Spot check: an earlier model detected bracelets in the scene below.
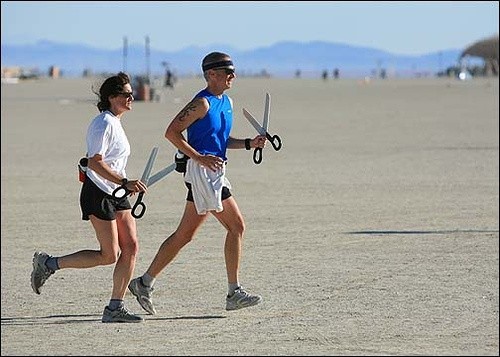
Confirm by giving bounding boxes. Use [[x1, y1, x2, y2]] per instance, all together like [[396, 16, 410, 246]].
[[244, 138, 250, 150]]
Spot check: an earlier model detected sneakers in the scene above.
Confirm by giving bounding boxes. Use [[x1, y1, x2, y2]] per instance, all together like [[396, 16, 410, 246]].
[[226, 285, 262, 311], [102, 301, 144, 322], [31, 250, 55, 295], [128, 277, 156, 315]]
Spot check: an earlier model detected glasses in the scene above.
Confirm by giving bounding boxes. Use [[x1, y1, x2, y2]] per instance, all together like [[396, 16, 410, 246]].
[[213, 68, 235, 75], [116, 92, 133, 98]]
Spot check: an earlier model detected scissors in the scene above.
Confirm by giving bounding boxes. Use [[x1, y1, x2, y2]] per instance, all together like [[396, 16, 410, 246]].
[[111, 147, 177, 219], [243, 93, 282, 164]]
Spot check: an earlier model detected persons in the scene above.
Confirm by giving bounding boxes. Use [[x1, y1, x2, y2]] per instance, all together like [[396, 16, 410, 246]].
[[30, 73, 147, 324], [127, 53, 266, 315]]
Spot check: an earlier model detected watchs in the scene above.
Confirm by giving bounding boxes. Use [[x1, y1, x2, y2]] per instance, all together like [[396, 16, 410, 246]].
[[122, 178, 127, 188]]
